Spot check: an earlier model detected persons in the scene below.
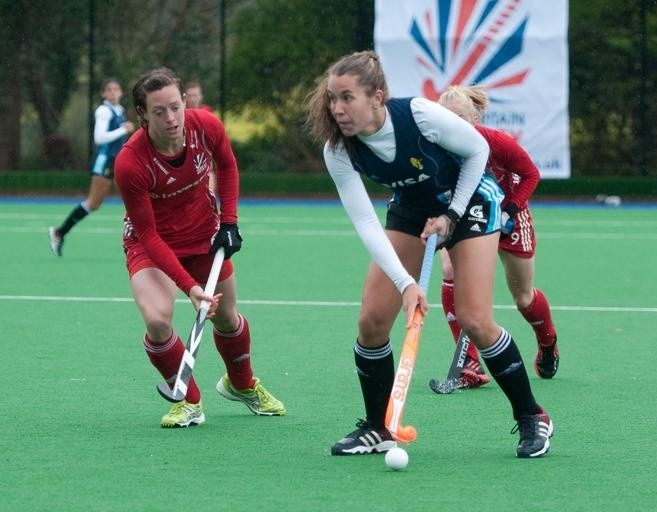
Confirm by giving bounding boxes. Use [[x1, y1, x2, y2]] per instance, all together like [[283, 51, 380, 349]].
[[304, 50, 554, 458], [184, 81, 217, 197], [48, 78, 135, 257], [114, 67, 286, 428], [435, 86, 560, 389]]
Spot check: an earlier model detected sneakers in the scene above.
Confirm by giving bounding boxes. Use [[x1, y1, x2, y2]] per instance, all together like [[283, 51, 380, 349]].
[[461, 356, 490, 388], [159, 397, 205, 428], [511, 407, 554, 454], [48, 226, 63, 257], [534, 333, 559, 379], [331, 419, 398, 456], [216, 372, 286, 415]]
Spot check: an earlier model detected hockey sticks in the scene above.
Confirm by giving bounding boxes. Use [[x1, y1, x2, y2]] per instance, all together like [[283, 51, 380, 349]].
[[384, 234, 437, 442], [154, 248, 226, 402], [429, 329, 470, 395]]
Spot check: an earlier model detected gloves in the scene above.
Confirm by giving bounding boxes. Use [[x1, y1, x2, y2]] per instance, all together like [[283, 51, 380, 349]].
[[501, 202, 519, 241], [209, 222, 243, 260]]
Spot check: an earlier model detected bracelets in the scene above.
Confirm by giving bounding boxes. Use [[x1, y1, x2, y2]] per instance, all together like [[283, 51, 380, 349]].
[[501, 201, 519, 219]]
